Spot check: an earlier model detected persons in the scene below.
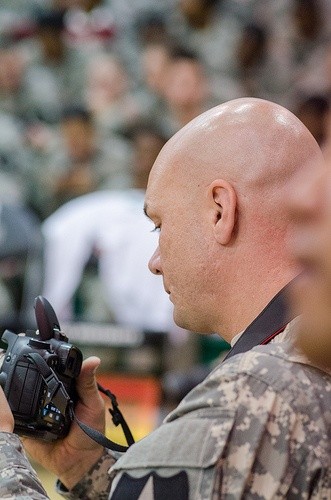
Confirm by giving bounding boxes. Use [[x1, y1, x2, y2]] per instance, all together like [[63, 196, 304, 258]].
[[0, 0, 331, 393], [0, 94, 331, 500]]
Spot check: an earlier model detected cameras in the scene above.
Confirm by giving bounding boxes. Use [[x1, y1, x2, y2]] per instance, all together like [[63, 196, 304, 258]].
[[0, 295, 83, 442]]
